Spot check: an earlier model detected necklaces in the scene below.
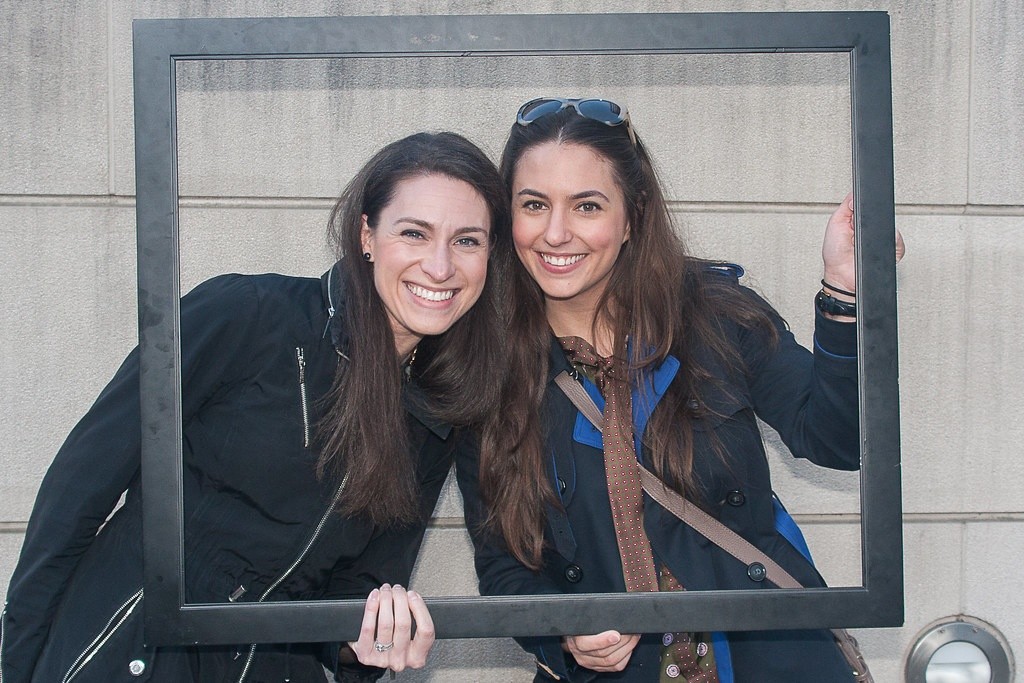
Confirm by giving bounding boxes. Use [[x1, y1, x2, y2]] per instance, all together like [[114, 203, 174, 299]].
[[408, 346, 418, 365]]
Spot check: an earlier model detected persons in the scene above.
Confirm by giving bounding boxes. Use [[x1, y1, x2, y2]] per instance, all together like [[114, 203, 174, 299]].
[[454, 98, 907, 683], [1, 129, 522, 683]]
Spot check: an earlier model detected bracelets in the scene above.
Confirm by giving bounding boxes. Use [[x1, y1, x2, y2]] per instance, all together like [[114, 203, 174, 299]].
[[816, 280, 859, 318]]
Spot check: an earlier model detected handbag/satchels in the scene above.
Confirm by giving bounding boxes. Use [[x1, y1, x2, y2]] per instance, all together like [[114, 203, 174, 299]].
[[830, 629, 875, 683]]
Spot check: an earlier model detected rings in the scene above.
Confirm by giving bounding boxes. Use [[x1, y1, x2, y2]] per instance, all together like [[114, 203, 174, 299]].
[[375, 638, 393, 652]]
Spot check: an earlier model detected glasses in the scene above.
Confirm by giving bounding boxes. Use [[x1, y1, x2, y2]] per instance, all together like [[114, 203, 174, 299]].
[[516, 97, 637, 152]]
